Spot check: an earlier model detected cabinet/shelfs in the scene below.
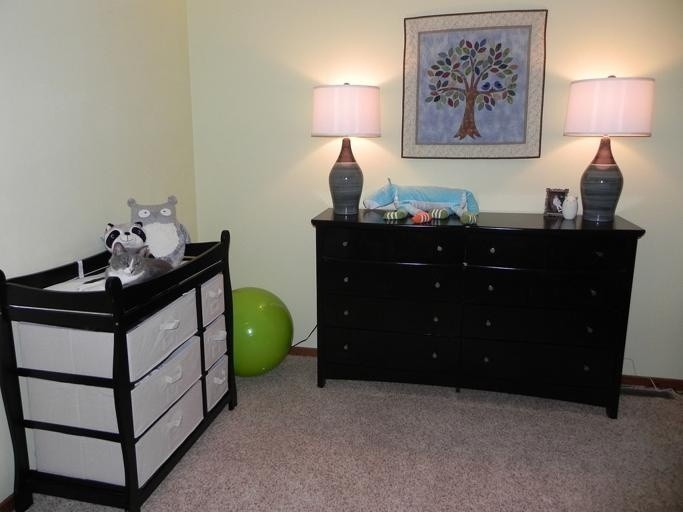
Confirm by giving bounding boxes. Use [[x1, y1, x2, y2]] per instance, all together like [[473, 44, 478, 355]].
[[313, 208, 464, 399], [0, 230, 238, 512], [465, 207, 647, 420]]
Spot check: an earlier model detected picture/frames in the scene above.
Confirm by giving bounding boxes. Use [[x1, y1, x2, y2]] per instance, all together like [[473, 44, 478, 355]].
[[401, 9, 548, 158], [544, 187, 568, 216]]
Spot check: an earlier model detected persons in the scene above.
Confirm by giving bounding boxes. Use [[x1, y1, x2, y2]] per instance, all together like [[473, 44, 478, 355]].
[[552, 194, 562, 212]]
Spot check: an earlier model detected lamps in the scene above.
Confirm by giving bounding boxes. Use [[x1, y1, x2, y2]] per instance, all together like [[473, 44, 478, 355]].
[[310, 83, 382, 215], [563, 74, 656, 222]]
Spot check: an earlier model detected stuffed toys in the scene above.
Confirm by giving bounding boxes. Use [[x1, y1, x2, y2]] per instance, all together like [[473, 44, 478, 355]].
[[363, 176, 479, 225], [128, 195, 190, 266], [103, 221, 155, 259]]
